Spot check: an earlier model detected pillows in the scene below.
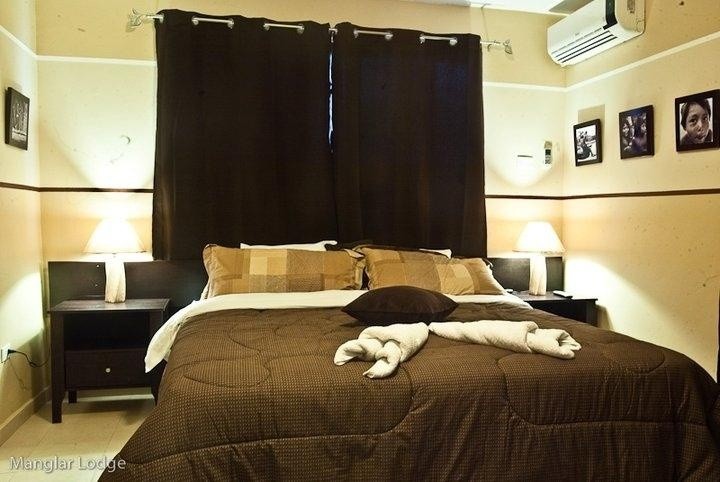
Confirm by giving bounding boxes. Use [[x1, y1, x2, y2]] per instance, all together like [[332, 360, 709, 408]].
[[323, 238, 454, 261], [235, 238, 342, 253], [339, 284, 461, 327], [354, 242, 505, 299], [200, 240, 365, 297]]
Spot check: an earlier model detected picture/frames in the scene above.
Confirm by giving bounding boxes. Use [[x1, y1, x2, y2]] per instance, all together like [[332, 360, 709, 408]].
[[571, 116, 603, 166], [619, 104, 654, 160], [5, 86, 30, 153], [674, 88, 719, 154]]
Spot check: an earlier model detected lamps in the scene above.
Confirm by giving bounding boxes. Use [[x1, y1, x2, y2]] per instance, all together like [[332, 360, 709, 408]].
[[83, 219, 149, 303], [511, 219, 568, 294]]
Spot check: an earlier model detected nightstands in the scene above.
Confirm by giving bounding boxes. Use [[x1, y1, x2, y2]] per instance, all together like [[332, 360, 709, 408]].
[[501, 283, 600, 327], [43, 295, 171, 427]]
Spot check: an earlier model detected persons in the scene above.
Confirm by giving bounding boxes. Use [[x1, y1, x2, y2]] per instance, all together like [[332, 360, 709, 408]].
[[621, 113, 647, 154], [679, 99, 713, 144], [574, 128, 596, 161]]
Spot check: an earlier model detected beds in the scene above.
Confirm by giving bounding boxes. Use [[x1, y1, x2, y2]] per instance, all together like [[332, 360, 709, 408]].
[[45, 238, 720, 482]]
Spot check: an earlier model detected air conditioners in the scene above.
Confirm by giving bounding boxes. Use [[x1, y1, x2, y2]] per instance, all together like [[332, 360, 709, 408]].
[[544, 0, 646, 70]]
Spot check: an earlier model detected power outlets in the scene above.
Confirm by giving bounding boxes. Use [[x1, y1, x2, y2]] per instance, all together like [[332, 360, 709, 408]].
[[0, 344, 10, 363]]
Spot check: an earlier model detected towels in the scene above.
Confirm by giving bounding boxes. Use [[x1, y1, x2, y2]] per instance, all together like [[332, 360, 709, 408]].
[[332, 320, 432, 384], [429, 318, 585, 362]]
